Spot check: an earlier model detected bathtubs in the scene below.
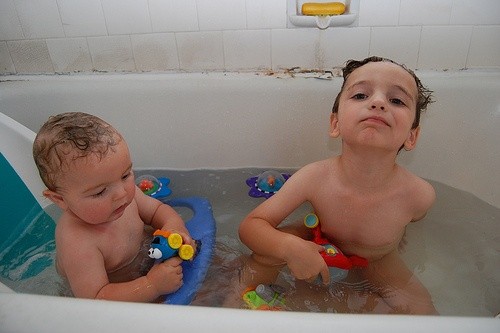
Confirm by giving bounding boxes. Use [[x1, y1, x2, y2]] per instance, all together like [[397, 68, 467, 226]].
[[0, 68, 499, 333]]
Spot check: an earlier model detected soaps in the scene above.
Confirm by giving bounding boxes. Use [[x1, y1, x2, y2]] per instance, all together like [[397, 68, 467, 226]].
[[302, 3, 346, 15]]
